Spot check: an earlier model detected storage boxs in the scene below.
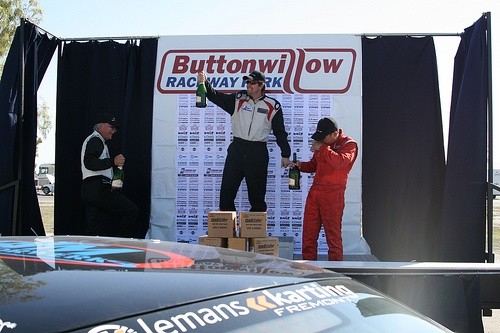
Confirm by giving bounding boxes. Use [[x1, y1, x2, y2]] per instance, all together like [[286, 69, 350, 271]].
[[199, 210, 278, 258]]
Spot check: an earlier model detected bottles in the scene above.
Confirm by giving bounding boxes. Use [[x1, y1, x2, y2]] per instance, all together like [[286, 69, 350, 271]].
[[288, 152, 300, 189], [196, 71, 207, 108], [111, 165, 125, 192]]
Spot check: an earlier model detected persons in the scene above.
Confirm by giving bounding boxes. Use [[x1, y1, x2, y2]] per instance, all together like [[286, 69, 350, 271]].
[[289, 117, 358, 261], [80, 112, 139, 234], [197, 71, 291, 252]]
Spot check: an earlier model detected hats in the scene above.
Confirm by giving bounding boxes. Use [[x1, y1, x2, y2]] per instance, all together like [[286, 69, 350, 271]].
[[91, 111, 121, 127], [311, 116, 338, 141], [243, 70, 265, 84]]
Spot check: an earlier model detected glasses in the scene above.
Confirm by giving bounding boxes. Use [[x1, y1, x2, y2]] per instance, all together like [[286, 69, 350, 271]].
[[246, 80, 259, 85]]
